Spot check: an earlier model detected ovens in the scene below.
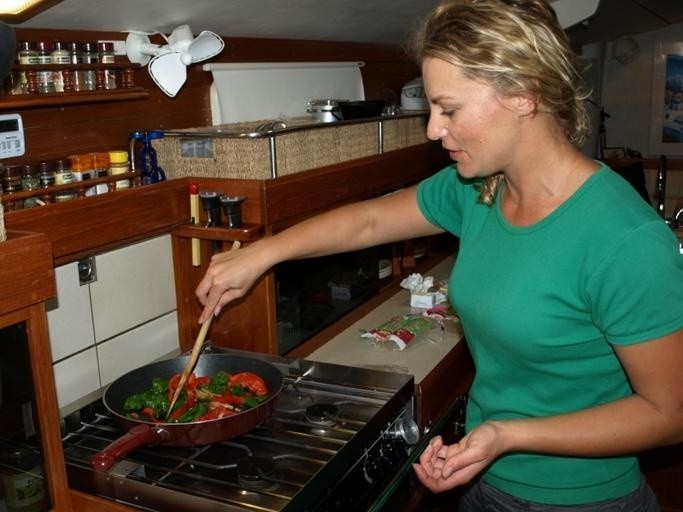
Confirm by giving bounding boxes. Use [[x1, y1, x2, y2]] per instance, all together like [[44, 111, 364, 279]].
[[306, 393, 466, 512]]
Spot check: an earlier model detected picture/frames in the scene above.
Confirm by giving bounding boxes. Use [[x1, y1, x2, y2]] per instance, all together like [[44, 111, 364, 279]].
[[601, 145, 626, 161], [645, 40, 683, 156]]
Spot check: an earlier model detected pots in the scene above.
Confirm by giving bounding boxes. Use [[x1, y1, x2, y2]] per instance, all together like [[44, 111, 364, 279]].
[[88, 353, 284, 472], [330, 100, 386, 121], [306, 99, 349, 123]]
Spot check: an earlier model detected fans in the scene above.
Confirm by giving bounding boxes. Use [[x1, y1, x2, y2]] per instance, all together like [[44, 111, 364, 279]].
[[118, 23, 224, 99]]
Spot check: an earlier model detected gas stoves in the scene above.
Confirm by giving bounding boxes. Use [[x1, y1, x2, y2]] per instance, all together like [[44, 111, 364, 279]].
[[24, 340, 413, 512]]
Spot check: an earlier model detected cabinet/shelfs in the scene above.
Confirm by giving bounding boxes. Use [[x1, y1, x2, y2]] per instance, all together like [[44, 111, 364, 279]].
[[0, 64, 190, 267], [1, 232, 71, 510], [171, 139, 458, 359], [417, 337, 477, 511]]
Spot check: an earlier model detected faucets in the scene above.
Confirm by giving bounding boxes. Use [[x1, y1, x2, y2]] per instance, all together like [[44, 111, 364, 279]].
[[655, 153, 675, 229]]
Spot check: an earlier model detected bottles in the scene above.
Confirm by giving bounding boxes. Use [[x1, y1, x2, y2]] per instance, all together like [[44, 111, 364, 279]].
[[3, 40, 134, 96], [0, 148, 131, 212], [0, 445, 49, 512]]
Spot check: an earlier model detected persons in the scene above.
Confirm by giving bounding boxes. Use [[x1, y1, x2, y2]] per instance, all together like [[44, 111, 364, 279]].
[[192, 0, 683, 511]]
